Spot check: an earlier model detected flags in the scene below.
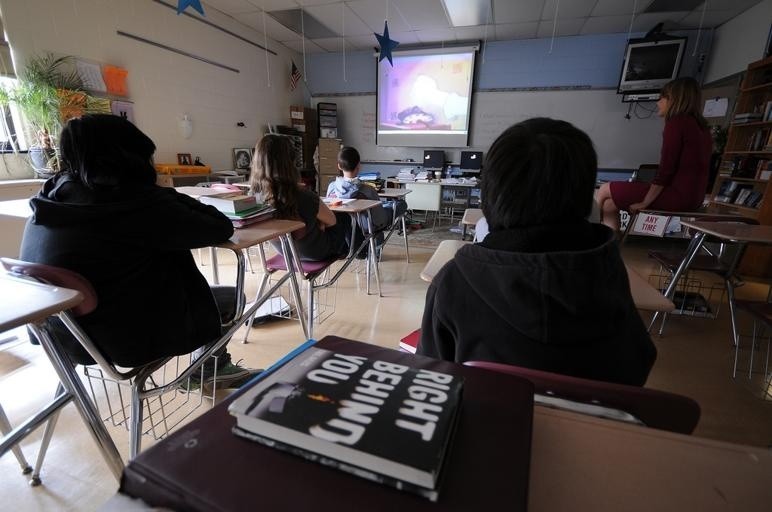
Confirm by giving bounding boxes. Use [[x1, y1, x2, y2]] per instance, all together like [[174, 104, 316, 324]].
[[290, 61, 301, 92]]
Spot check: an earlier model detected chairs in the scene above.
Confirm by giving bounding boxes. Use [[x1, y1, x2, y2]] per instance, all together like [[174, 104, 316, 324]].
[[734, 287, 769, 382], [0, 175, 772, 300], [462, 361, 700, 431], [240, 232, 349, 341], [0, 258, 218, 486]]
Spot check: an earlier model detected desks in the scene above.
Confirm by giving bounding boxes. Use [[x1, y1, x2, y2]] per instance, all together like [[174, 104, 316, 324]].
[[153, 163, 211, 188], [0, 273, 123, 485], [420, 238, 675, 334], [228, 219, 307, 339], [100, 405, 772, 510]]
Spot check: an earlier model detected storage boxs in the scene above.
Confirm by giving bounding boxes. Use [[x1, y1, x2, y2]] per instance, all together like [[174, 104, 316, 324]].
[[290, 104, 316, 120], [291, 120, 317, 134], [319, 138, 343, 175], [300, 134, 315, 143]]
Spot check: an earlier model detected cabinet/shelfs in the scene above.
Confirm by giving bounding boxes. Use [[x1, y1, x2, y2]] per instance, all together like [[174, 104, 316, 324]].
[[710, 54, 772, 281]]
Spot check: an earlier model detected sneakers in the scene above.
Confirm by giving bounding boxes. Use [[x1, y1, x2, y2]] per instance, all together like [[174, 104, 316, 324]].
[[177, 359, 264, 394]]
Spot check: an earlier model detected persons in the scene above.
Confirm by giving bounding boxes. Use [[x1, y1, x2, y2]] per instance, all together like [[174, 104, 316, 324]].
[[247, 133, 369, 263], [326, 146, 393, 262], [416, 117, 658, 387], [596, 77, 713, 240], [20, 113, 265, 393], [237, 151, 250, 171]]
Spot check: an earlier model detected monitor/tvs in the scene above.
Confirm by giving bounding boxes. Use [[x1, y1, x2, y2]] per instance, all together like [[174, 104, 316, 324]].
[[460, 150, 484, 174], [616, 36, 689, 93], [424, 149, 445, 170]]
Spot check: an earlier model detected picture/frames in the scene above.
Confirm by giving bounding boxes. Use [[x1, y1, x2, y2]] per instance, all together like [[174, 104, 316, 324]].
[[232, 147, 253, 171], [177, 153, 193, 165]]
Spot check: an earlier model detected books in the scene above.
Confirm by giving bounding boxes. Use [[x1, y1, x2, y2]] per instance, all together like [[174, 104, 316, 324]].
[[400, 328, 421, 354], [396, 168, 432, 184], [227, 347, 466, 504], [198, 193, 277, 229], [713, 100, 772, 208]]
